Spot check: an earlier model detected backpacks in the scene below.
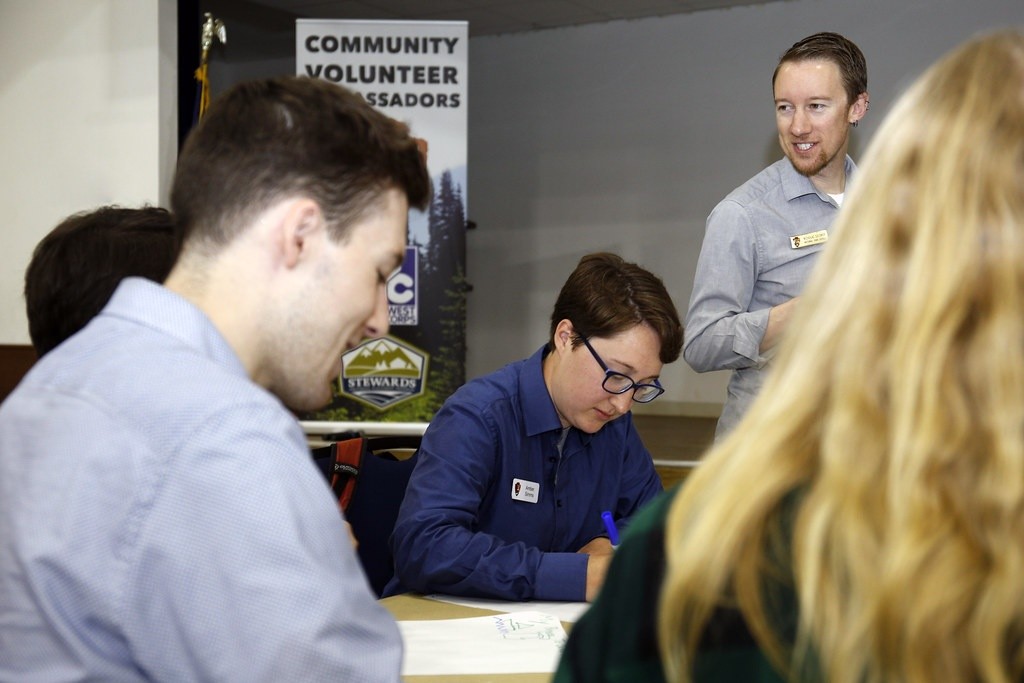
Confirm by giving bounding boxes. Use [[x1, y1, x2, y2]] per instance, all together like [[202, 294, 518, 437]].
[[310, 439, 419, 600]]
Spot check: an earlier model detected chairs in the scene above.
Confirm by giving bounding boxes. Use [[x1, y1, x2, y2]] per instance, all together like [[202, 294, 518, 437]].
[[313, 436, 423, 598]]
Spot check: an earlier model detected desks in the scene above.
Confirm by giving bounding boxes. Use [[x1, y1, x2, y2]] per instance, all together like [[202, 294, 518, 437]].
[[376, 593, 591, 683]]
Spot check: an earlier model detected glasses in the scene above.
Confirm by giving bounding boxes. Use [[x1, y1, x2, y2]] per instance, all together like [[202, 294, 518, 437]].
[[584, 339, 665, 403]]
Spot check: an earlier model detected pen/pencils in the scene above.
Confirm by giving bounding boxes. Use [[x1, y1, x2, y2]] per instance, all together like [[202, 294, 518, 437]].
[[601, 511, 621, 552]]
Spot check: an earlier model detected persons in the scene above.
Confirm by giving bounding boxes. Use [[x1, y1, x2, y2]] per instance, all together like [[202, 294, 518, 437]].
[[23, 207, 181, 356], [0, 77, 429, 683], [382, 253, 685, 602], [681, 32, 869, 445], [552, 20, 1024, 683]]
